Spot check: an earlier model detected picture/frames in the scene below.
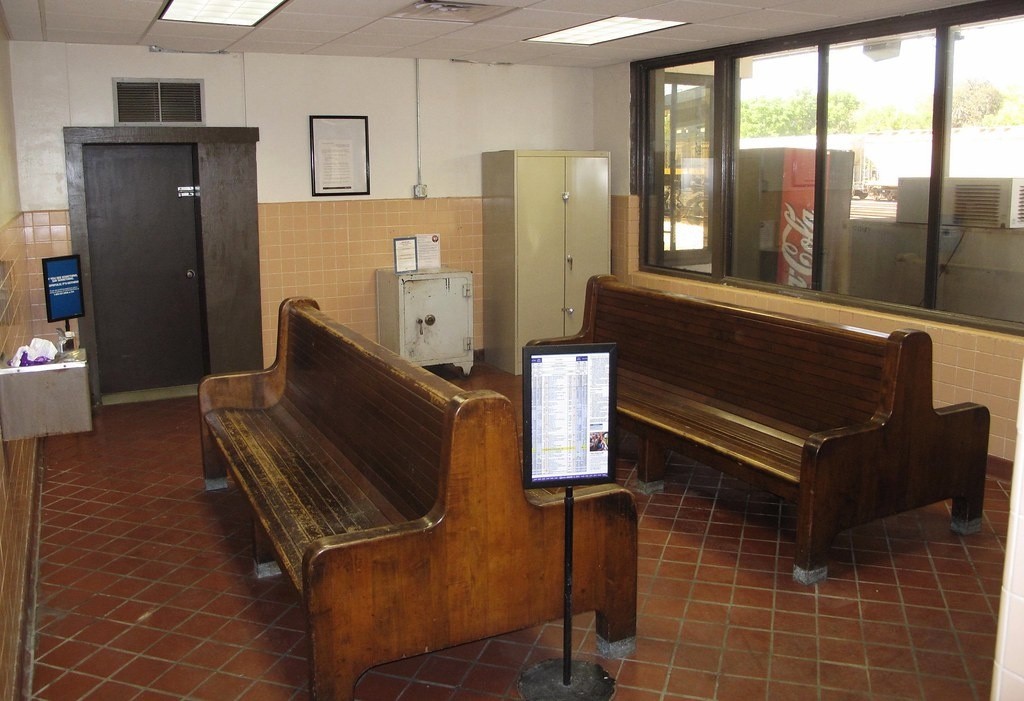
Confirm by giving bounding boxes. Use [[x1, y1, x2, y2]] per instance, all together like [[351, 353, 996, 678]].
[[309, 115, 370, 196]]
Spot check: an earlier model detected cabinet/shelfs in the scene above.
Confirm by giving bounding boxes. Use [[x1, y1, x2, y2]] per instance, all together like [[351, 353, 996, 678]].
[[375, 267, 476, 378], [481, 150, 612, 375]]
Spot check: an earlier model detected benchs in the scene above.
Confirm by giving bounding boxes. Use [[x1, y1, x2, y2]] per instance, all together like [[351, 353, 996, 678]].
[[523, 274, 990, 589], [198, 297, 639, 695]]
